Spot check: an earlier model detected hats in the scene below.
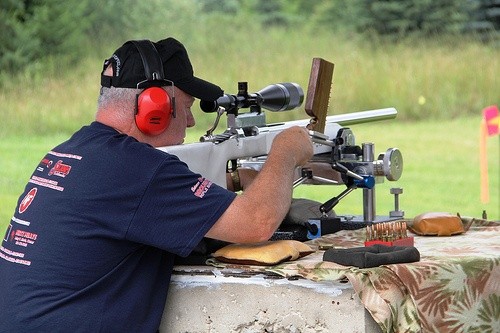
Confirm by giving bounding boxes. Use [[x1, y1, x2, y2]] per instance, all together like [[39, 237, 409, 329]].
[[100, 37, 224, 101]]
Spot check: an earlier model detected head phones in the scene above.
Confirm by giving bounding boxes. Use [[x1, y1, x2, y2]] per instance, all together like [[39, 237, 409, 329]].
[[129, 39, 176, 137]]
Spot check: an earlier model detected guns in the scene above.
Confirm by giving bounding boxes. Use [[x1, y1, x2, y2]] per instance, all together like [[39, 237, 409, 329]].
[[155, 81, 397, 190]]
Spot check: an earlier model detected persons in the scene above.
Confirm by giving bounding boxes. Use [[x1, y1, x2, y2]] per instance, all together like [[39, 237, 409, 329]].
[[0, 36, 312, 332]]
